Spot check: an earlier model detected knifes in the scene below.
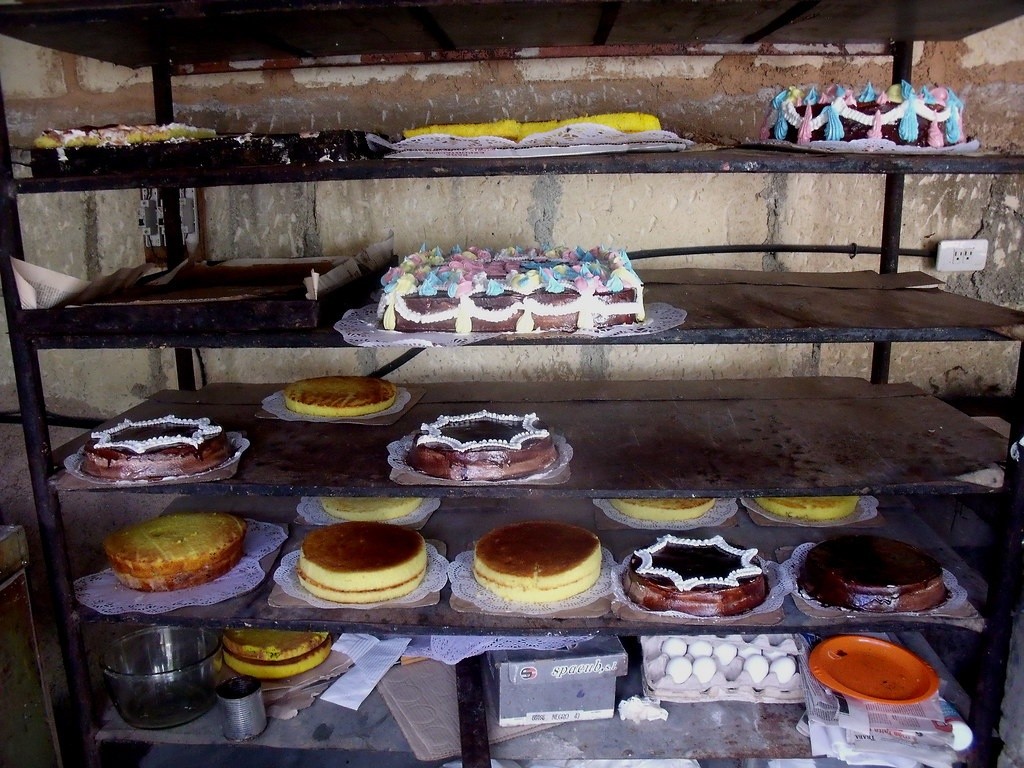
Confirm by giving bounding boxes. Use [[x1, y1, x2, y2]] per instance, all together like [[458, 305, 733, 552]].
[[301, 655, 430, 691]]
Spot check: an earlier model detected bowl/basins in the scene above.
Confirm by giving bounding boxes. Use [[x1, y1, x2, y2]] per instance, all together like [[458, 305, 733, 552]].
[[101, 625, 222, 729]]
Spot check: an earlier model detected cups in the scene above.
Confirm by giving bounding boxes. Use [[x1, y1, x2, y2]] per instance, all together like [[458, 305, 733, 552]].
[[216, 676, 267, 740]]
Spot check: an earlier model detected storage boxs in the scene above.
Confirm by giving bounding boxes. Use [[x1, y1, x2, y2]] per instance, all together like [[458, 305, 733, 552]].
[[485, 635, 628, 728]]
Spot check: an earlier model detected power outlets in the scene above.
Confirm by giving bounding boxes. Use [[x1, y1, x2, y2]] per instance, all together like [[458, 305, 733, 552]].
[[936, 239, 988, 272]]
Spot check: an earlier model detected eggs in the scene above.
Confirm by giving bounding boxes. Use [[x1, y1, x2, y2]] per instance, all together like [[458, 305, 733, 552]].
[[662, 638, 796, 683]]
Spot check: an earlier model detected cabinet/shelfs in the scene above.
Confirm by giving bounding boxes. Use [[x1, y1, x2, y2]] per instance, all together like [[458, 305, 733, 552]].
[[0, 526, 64, 768], [0, 0, 1024, 768]]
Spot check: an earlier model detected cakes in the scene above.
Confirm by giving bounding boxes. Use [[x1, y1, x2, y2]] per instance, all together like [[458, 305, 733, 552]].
[[283, 375, 396, 416], [621, 533, 769, 616], [294, 496, 427, 604], [607, 498, 716, 521], [33, 120, 216, 148], [80, 414, 232, 479], [798, 534, 949, 614], [221, 626, 332, 679], [472, 520, 602, 602], [371, 251, 647, 333], [102, 511, 249, 592], [760, 79, 967, 148], [404, 408, 558, 481], [755, 496, 859, 521], [394, 112, 663, 134]]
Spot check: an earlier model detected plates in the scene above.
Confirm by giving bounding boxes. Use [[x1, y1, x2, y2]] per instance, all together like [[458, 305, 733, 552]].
[[809, 635, 939, 704]]
